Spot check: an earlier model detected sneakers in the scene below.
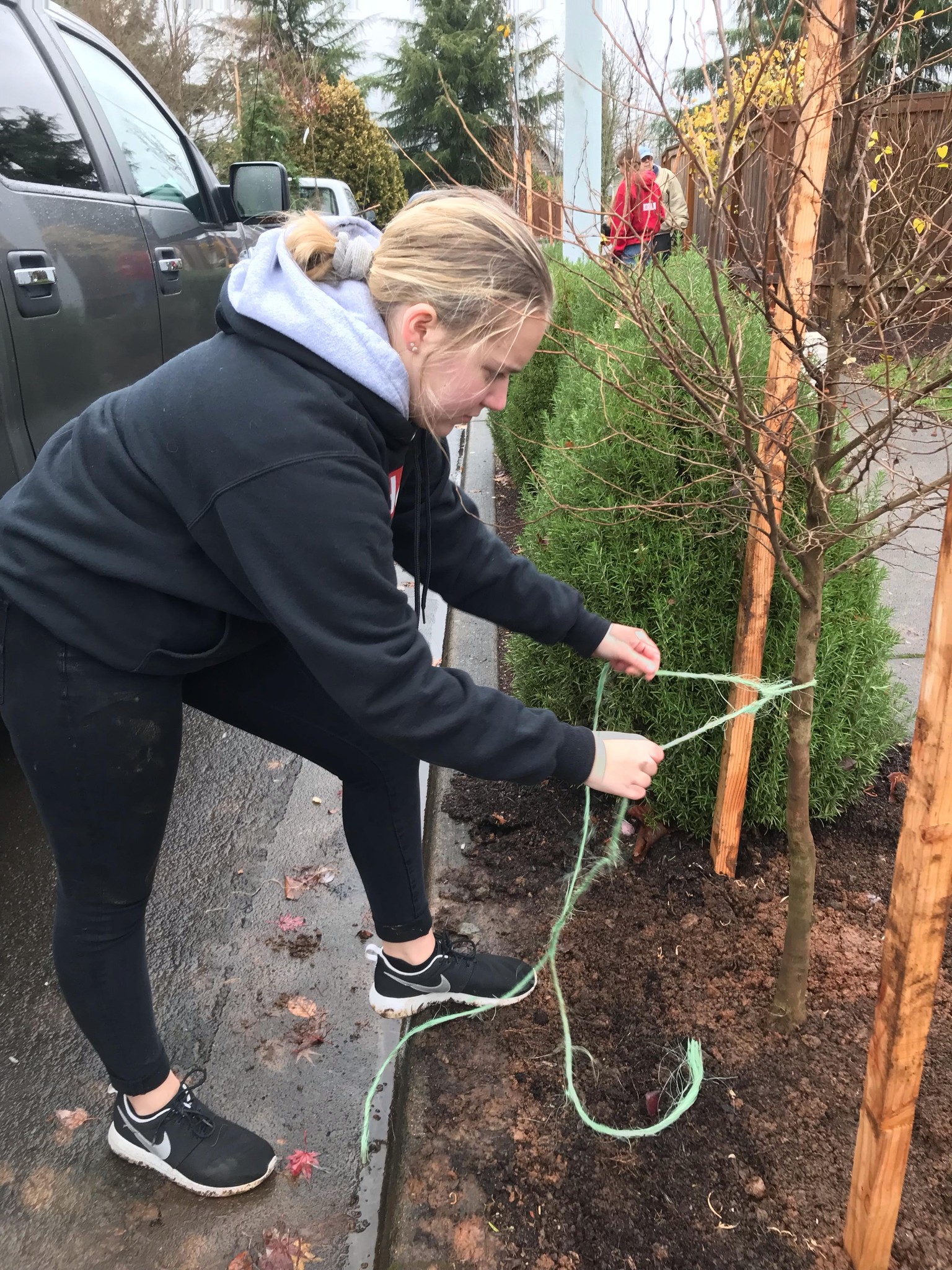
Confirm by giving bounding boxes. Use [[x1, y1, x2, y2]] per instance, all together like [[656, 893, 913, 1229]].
[[364, 928, 537, 1019], [108, 1067, 276, 1197]]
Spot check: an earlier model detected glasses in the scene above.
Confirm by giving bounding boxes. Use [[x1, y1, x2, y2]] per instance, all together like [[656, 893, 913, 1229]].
[[641, 156, 653, 162]]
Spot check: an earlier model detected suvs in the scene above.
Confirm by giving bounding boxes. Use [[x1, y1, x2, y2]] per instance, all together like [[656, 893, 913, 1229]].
[[0, 1, 289, 496]]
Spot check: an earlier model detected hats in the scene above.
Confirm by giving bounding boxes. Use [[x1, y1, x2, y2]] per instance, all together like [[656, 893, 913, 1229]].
[[638, 145, 653, 159]]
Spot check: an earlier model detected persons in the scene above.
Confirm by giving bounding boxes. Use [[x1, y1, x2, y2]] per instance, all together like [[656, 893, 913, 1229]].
[[0, 187, 667, 1198], [601, 146, 690, 328]]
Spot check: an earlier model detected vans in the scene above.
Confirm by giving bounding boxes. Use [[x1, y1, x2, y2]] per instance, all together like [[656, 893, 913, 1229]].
[[287, 177, 365, 221]]
